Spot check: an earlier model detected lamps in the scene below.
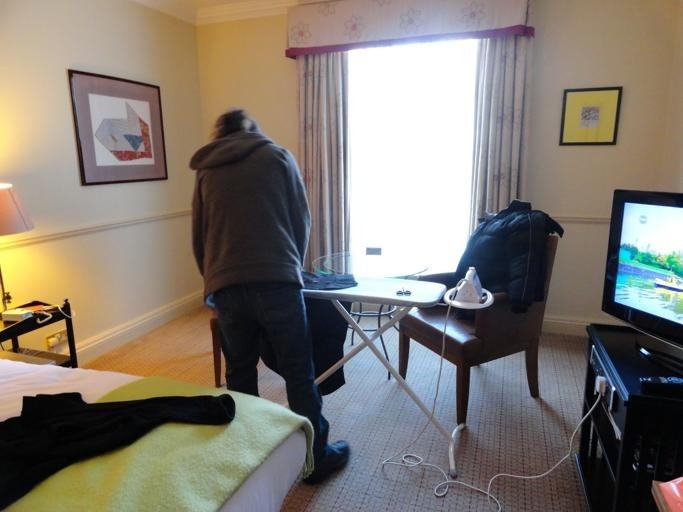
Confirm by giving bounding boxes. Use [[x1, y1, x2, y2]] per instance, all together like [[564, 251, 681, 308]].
[[399, 216, 559, 431], [208, 316, 227, 387]]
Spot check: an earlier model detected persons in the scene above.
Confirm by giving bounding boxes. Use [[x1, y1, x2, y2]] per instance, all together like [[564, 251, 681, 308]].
[[186, 109, 350, 485]]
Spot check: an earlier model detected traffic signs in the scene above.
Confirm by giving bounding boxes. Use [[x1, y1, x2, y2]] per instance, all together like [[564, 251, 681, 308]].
[[46, 328, 68, 349]]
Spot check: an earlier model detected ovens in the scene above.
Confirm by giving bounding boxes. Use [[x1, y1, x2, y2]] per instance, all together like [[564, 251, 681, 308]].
[[1, 357, 316, 511]]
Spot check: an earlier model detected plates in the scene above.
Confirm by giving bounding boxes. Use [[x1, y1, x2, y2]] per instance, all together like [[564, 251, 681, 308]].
[[574, 323, 683, 512]]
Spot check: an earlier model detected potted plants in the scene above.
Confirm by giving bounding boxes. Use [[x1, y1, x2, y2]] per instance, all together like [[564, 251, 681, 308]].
[[68, 68, 168, 185], [557, 85, 622, 145]]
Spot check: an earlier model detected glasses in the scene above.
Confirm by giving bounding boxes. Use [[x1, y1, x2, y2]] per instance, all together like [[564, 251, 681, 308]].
[[0, 181, 37, 319]]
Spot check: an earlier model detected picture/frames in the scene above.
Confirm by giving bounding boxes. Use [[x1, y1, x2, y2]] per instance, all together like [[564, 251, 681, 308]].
[[205, 271, 467, 478]]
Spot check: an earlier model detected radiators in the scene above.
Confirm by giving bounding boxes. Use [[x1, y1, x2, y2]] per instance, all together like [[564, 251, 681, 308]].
[[639, 376, 683, 386]]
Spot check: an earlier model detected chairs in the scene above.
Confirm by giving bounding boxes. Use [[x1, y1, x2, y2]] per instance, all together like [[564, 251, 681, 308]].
[[303, 441, 348, 485]]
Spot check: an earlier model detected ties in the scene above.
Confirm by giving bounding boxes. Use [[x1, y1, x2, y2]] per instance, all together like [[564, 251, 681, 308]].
[[599, 190, 683, 375]]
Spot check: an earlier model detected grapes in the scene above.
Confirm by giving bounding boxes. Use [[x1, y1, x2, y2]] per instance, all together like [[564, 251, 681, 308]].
[[0, 299, 78, 372]]
